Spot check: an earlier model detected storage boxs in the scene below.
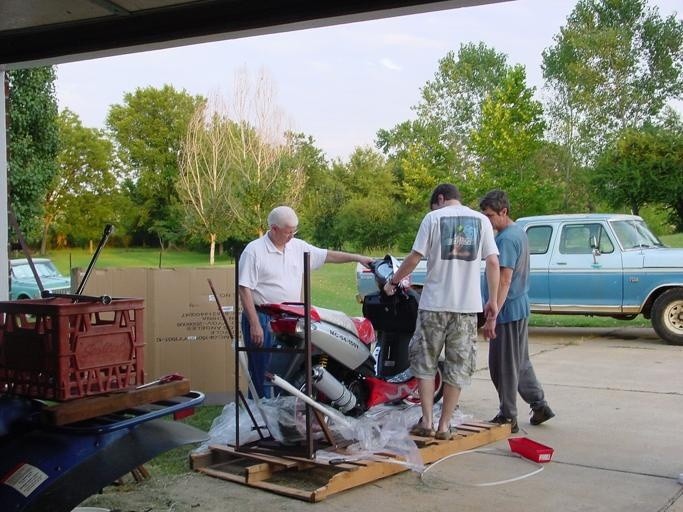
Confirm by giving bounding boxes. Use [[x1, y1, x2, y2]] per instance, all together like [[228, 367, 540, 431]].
[[68, 267, 256, 407]]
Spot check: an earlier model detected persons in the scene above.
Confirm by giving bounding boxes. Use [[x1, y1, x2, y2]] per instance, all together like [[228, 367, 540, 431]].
[[234, 203, 377, 443], [382, 182, 501, 441], [476, 188, 556, 435]]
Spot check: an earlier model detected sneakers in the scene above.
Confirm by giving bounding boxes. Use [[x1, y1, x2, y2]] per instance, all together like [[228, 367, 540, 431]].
[[488, 413, 519, 433], [530, 406, 555, 425]]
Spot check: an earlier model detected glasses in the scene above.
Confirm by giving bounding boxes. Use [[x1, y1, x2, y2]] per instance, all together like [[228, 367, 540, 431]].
[[284, 229, 298, 235]]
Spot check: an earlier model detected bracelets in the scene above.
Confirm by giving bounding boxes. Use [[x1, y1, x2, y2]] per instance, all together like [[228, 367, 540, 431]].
[[388, 274, 399, 286]]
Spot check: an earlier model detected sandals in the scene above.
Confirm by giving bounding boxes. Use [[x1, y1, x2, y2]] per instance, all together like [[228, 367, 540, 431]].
[[411, 416, 451, 439]]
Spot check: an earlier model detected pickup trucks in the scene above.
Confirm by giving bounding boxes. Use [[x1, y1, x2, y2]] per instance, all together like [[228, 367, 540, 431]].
[[356, 213, 683, 345]]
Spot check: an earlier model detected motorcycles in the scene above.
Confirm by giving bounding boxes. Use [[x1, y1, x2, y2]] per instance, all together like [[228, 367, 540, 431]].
[[261, 252, 443, 451]]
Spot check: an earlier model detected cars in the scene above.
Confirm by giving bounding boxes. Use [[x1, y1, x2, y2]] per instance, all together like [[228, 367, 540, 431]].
[[8, 258, 71, 319]]
